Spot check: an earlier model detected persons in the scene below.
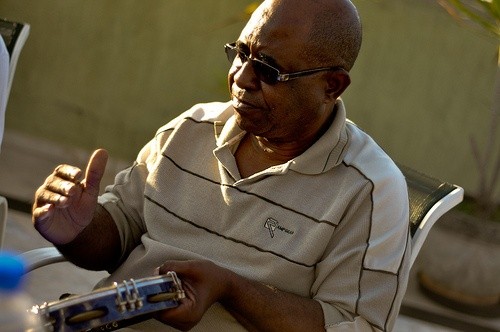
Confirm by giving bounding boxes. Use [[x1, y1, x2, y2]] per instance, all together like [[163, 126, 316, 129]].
[[30, 1, 411, 331]]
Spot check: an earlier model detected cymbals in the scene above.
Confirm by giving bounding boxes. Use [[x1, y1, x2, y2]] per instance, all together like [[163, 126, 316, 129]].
[[17, 271, 186, 331]]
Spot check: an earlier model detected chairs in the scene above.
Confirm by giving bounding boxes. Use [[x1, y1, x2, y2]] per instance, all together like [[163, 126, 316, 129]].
[[1, 17, 30, 251], [7, 160, 464, 329]]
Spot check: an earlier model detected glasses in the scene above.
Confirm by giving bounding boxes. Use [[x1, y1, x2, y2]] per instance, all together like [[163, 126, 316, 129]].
[[224, 41, 341, 86]]
